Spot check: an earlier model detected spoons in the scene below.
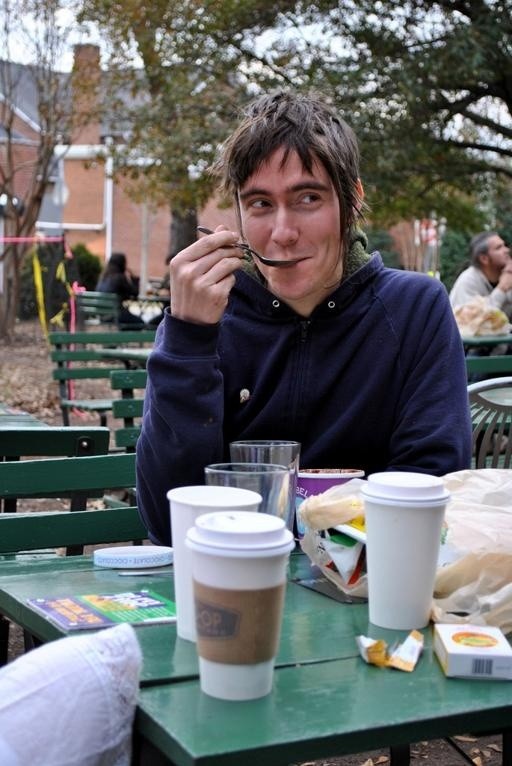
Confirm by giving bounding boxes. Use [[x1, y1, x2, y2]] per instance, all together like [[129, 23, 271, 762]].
[[196, 225, 298, 267]]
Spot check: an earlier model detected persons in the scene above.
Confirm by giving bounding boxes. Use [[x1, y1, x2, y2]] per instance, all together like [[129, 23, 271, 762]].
[[144, 253, 178, 325], [94, 252, 145, 332], [133, 85, 472, 549], [444, 228, 511, 339]]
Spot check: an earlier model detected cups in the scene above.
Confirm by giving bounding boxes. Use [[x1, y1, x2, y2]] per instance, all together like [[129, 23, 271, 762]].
[[204, 462, 290, 529], [229, 439, 302, 532], [166, 485, 263, 643], [358, 470, 452, 631], [184, 510, 296, 702]]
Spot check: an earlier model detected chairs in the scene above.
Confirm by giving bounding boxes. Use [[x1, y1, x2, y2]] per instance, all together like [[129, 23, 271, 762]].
[[50, 330, 159, 426], [108, 369, 150, 454], [73, 288, 156, 350], [463, 375, 512, 469], [1, 453, 151, 559]]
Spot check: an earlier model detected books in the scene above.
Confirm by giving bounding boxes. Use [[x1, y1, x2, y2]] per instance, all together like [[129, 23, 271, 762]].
[[26, 585, 177, 631]]
